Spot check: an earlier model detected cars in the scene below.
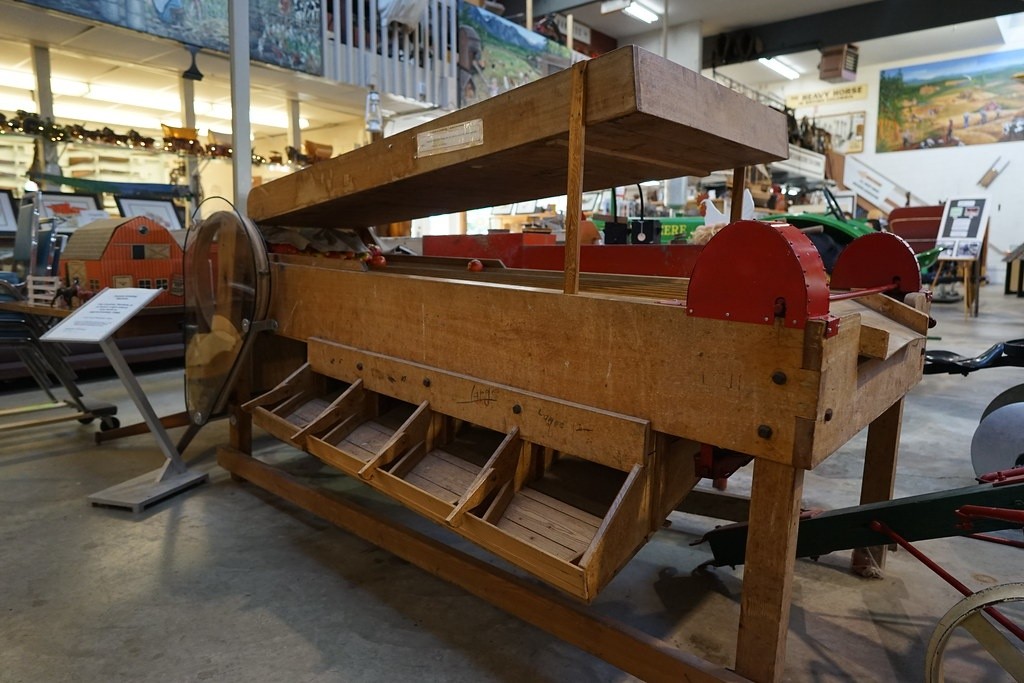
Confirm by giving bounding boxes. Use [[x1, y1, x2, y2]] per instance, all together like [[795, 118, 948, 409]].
[[627, 188, 948, 275]]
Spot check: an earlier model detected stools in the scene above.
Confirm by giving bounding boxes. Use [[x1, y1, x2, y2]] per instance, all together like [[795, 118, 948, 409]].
[[930, 256, 975, 317]]
